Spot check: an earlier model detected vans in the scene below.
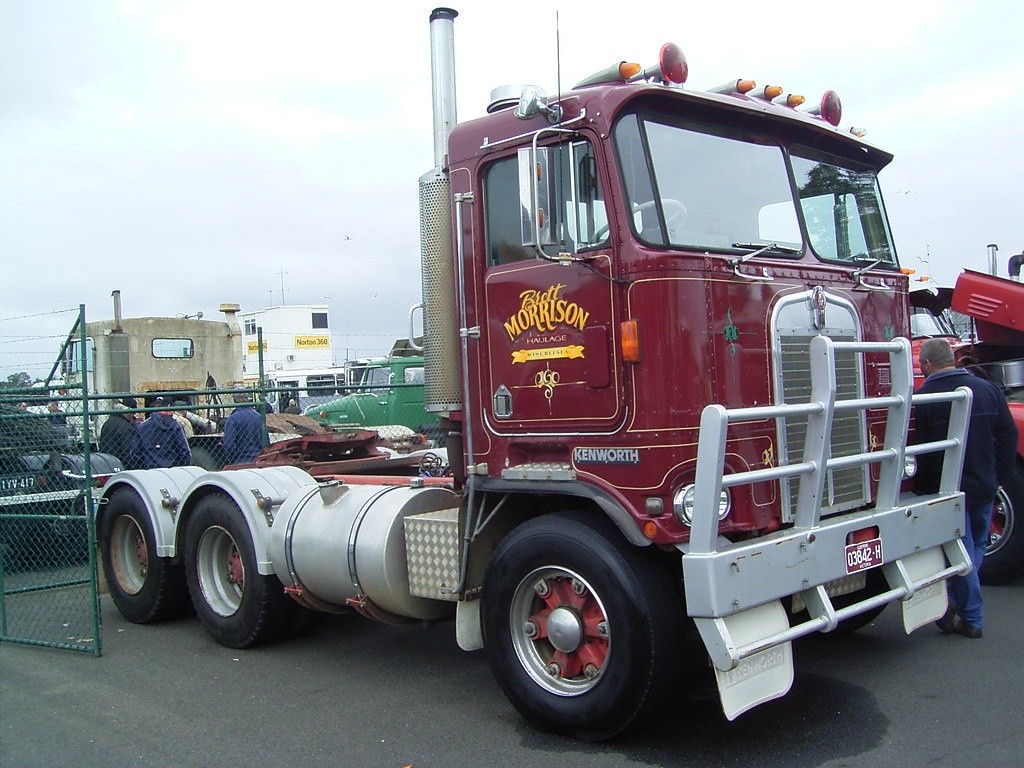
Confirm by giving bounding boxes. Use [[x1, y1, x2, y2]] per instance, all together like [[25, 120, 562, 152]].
[[299, 357, 443, 433]]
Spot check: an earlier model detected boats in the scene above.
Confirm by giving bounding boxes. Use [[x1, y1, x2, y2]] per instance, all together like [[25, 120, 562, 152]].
[[234, 304, 387, 415]]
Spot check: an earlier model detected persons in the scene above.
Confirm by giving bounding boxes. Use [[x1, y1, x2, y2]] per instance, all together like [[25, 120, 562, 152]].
[[255, 396, 274, 414], [284, 399, 300, 415], [223, 387, 263, 466], [910, 338, 1019, 638], [47, 401, 67, 427], [20, 402, 27, 410], [100, 396, 195, 470]]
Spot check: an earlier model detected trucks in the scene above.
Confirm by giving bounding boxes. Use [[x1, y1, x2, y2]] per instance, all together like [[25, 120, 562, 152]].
[[60, 288, 243, 451]]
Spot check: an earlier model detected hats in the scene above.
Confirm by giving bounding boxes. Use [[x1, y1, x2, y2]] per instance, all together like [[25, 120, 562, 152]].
[[117, 397, 137, 409], [156, 396, 175, 406], [230, 387, 248, 398]]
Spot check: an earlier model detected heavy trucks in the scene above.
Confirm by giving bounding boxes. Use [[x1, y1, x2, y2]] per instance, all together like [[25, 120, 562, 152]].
[[94, 7, 977, 744], [0, 404, 126, 561], [909, 244, 1024, 587]]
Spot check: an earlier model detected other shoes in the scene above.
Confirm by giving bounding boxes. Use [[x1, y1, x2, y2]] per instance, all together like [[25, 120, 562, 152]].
[[935, 601, 957, 633], [952, 616, 982, 638]]
